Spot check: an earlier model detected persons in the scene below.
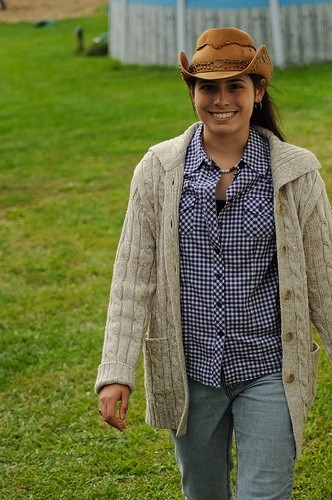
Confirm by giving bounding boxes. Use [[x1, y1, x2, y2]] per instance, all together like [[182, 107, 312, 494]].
[[93, 27, 331, 500]]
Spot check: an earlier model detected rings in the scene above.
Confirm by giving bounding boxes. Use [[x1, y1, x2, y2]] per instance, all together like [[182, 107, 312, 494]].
[[98, 409, 102, 414]]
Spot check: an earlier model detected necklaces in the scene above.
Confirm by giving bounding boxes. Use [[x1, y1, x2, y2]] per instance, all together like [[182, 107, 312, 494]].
[[215, 159, 242, 174]]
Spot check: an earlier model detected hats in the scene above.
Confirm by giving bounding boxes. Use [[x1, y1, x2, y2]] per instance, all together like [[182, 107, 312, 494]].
[[179, 27, 273, 88]]
